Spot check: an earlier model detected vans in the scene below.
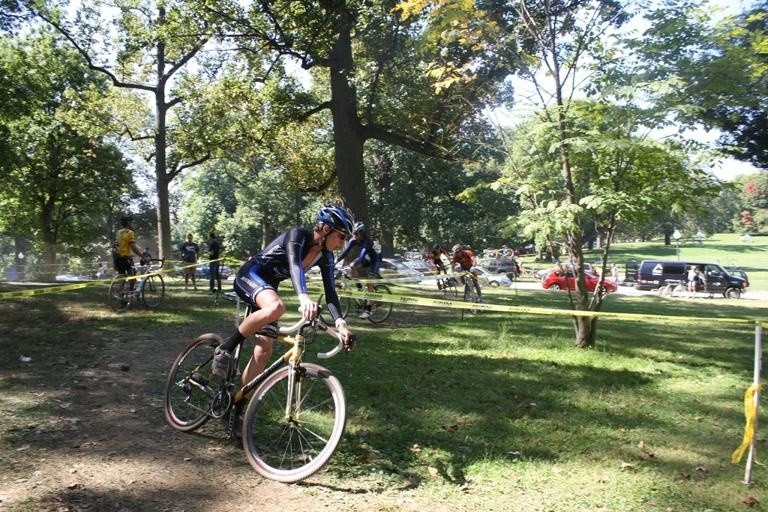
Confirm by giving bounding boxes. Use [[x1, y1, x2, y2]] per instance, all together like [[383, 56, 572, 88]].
[[638, 260, 747, 299], [479, 258, 520, 280]]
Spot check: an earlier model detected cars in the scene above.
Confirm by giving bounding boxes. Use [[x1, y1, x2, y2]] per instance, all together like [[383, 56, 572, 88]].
[[174, 258, 231, 279], [537, 263, 617, 296], [515, 244, 535, 255], [470, 266, 511, 286], [347, 257, 425, 284]]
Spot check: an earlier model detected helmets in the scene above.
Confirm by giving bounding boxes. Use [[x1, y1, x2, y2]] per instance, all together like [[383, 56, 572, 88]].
[[318, 206, 353, 236], [452, 244, 462, 253], [434, 243, 441, 250], [353, 222, 365, 234], [121, 216, 133, 225]]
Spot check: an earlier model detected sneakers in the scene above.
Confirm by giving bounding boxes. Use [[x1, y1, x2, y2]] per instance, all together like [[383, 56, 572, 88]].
[[212, 344, 231, 378], [222, 410, 243, 438], [359, 311, 373, 319], [477, 294, 482, 302]]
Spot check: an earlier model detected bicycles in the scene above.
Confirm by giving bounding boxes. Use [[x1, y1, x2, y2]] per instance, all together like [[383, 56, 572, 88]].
[[107, 258, 166, 313], [487, 245, 514, 260], [318, 267, 393, 324], [435, 265, 458, 297], [520, 267, 537, 281], [163, 292, 356, 483], [658, 278, 691, 300], [460, 272, 485, 314]]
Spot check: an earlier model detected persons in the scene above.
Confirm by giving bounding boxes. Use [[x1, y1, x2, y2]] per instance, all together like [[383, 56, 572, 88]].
[[333, 221, 381, 320], [610, 263, 619, 292], [140, 247, 151, 274], [97, 263, 105, 280], [423, 242, 482, 303], [212, 205, 355, 439], [202, 232, 222, 293], [180, 234, 200, 291], [113, 215, 150, 298], [687, 266, 697, 299]]
